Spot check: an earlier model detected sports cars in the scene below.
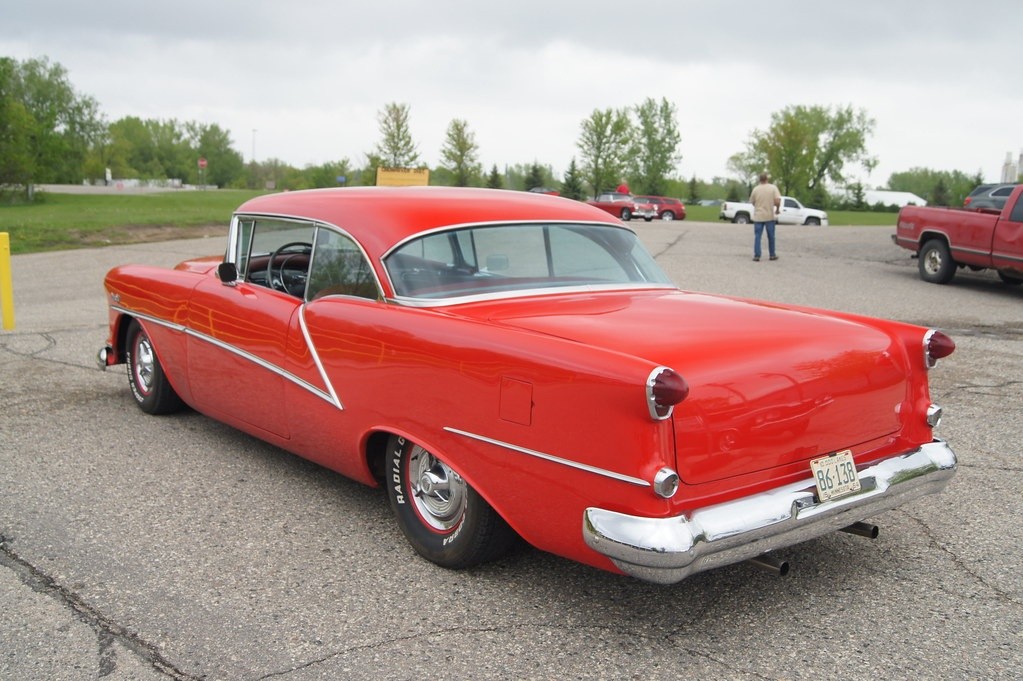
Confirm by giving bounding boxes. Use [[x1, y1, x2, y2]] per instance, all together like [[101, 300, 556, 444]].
[[94, 184, 960, 571]]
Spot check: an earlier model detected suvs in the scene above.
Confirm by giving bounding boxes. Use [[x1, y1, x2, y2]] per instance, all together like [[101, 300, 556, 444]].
[[961, 182, 1023, 216]]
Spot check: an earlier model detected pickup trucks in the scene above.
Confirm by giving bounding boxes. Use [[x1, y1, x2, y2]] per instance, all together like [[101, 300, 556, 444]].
[[719, 195, 830, 226]]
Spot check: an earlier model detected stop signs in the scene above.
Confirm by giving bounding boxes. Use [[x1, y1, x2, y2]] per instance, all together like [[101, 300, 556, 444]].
[[198, 158, 208, 169]]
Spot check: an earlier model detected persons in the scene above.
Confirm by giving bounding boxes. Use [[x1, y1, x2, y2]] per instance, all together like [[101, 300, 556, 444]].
[[749, 174, 781, 262]]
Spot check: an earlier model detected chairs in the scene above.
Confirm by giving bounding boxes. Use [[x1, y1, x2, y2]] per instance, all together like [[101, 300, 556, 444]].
[[311, 283, 377, 302]]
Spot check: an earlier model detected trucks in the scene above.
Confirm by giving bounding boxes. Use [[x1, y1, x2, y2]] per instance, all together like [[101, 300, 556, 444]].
[[890, 182, 1023, 284]]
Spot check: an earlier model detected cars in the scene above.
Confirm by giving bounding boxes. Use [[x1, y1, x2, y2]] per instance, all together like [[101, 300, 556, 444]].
[[634, 194, 686, 221], [581, 193, 658, 221], [529, 186, 560, 199]]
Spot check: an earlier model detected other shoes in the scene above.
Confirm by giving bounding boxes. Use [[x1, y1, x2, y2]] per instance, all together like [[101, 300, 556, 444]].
[[752, 256, 760, 261], [770, 256, 778, 260]]
